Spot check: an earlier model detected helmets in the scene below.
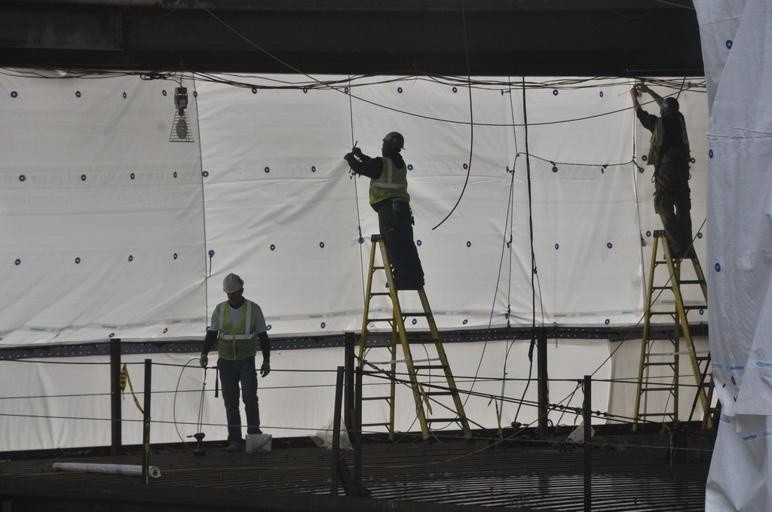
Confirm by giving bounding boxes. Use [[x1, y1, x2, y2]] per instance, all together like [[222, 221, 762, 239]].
[[222, 272, 243, 294], [662, 97, 679, 112], [383, 131, 404, 147]]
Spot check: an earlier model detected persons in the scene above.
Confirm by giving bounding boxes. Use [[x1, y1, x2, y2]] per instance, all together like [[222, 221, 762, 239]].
[[198, 271, 273, 452], [342, 130, 428, 290], [628, 81, 697, 260]]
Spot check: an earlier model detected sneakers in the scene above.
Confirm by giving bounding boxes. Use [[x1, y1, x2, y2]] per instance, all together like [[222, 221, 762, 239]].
[[226, 441, 241, 451]]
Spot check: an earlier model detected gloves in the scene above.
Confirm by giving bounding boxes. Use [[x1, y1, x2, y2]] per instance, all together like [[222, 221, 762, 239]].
[[260, 361, 270, 377], [200, 353, 208, 367], [352, 146, 361, 157], [344, 152, 354, 161]]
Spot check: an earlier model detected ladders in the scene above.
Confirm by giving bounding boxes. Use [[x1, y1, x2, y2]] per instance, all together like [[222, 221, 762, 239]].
[[632, 230, 720, 433], [355, 234, 472, 442]]
